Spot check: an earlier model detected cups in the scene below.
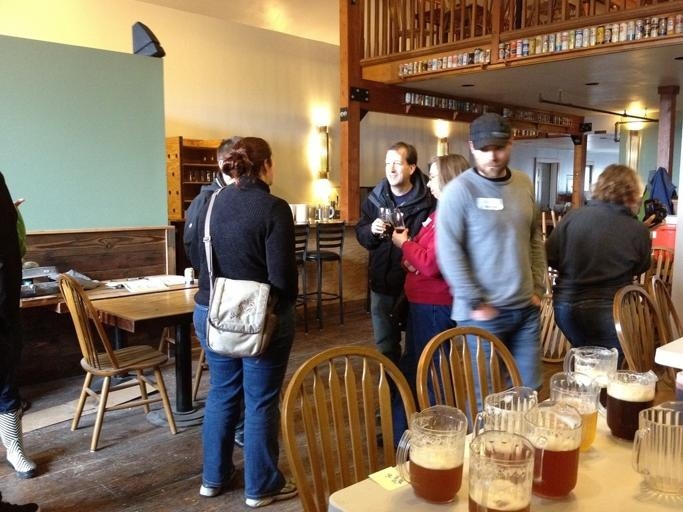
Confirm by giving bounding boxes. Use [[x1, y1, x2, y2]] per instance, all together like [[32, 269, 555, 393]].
[[287, 202, 335, 226], [598, 370, 657, 443], [524, 400, 583, 500], [467, 430, 536, 511], [396, 400, 466, 501], [376, 204, 412, 243], [560, 344, 619, 384], [545, 370, 600, 452], [185, 168, 221, 183], [634, 407, 683, 496], [473, 385, 537, 436]]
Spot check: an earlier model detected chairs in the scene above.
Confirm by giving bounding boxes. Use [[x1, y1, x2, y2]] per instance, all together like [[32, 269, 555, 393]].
[[649, 275, 682, 381], [612, 283, 677, 385], [57, 274, 177, 452], [306, 220, 347, 331], [416, 326, 522, 435], [281, 344, 417, 512], [640, 247, 674, 285], [293, 223, 310, 336], [539, 210, 572, 362]]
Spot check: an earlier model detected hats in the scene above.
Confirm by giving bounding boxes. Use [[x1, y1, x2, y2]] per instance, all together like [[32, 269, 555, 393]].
[[469, 113, 510, 149]]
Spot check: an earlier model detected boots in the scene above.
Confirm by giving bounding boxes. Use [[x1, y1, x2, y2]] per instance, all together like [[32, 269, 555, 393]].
[[0, 407, 37, 479]]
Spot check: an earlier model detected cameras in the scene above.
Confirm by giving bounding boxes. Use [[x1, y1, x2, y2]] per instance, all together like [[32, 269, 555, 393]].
[[642, 199, 667, 227]]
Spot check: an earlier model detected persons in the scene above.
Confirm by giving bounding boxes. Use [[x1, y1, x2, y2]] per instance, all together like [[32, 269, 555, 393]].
[[433, 113, 548, 434], [545, 164, 656, 369], [356, 142, 437, 425], [377, 154, 471, 448], [0, 171, 38, 479], [199, 136, 299, 508], [0, 491, 41, 512], [183, 136, 245, 448]]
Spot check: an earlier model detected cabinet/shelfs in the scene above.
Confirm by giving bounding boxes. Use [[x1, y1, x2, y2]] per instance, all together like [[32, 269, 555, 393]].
[[166, 137, 223, 222]]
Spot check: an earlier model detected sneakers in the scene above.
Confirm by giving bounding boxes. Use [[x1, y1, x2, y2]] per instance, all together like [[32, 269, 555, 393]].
[[0, 502, 40, 512], [232, 426, 244, 448], [199, 465, 235, 497], [245, 477, 298, 508]]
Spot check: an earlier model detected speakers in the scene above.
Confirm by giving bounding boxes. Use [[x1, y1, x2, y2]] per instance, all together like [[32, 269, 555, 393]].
[[132, 22, 165, 58], [571, 132, 582, 145]]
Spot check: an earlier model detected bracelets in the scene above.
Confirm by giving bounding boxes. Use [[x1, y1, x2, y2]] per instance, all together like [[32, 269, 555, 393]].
[[469, 297, 484, 309]]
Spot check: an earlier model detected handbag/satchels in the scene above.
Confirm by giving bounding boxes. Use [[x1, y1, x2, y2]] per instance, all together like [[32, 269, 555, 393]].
[[206, 278, 276, 357]]
